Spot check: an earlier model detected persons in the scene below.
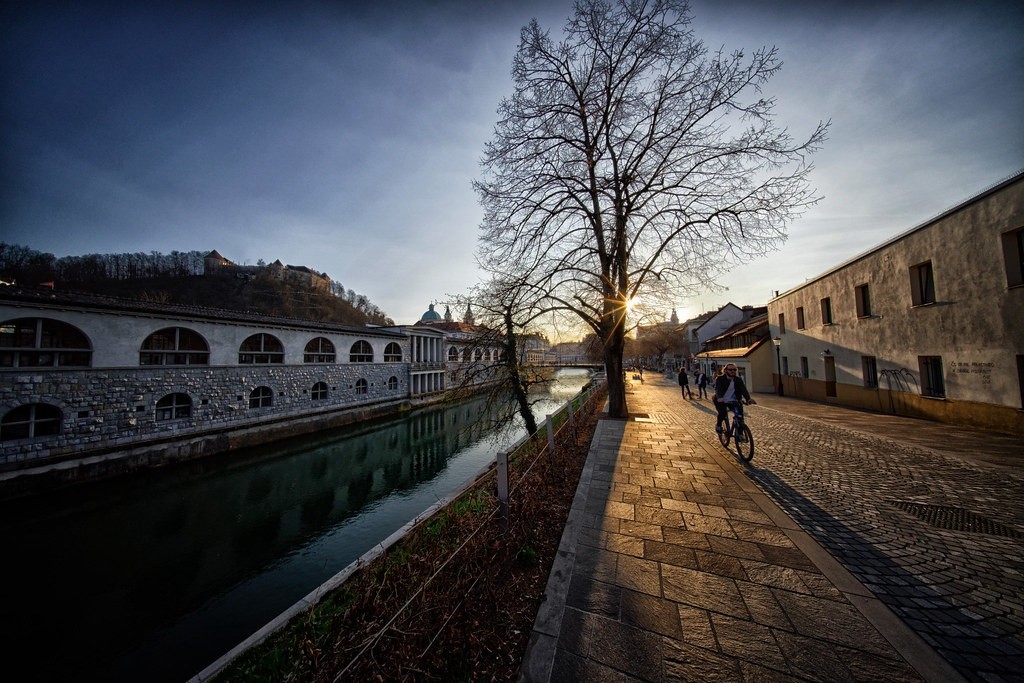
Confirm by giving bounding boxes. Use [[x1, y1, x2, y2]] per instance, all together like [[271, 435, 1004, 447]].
[[711, 363, 757, 442], [694, 369, 710, 400], [678, 367, 693, 400]]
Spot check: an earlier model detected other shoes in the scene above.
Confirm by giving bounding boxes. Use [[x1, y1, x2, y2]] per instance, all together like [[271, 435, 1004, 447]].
[[739, 435, 745, 441], [716, 425, 722, 435]]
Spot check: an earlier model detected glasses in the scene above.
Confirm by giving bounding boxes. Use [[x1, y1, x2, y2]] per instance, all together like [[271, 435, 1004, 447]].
[[727, 368, 737, 371]]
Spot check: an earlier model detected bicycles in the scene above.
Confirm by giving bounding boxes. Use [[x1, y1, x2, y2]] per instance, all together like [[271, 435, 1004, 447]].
[[718, 398, 755, 462], [623, 380, 634, 391]]
